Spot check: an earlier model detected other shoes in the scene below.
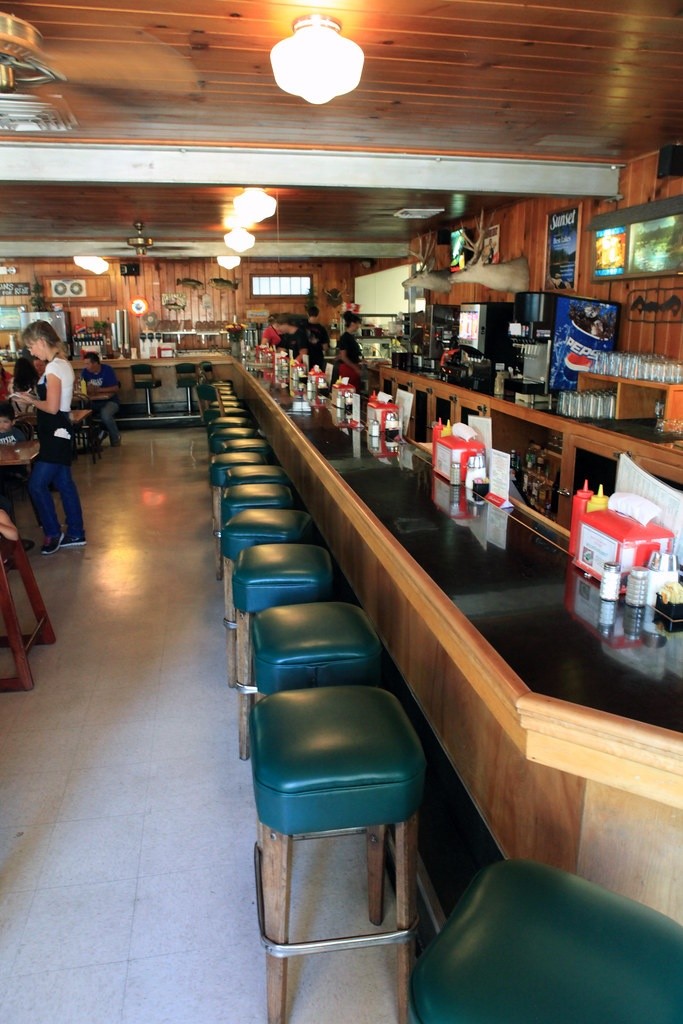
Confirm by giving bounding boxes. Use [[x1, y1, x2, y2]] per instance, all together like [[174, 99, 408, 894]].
[[111, 439, 120, 446]]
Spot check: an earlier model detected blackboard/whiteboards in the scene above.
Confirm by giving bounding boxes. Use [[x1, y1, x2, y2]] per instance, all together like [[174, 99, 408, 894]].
[[0, 282, 30, 296]]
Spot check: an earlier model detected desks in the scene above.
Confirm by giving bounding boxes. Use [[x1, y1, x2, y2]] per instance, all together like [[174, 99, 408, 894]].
[[72, 392, 117, 456], [0, 439, 40, 552], [69, 409, 102, 464]]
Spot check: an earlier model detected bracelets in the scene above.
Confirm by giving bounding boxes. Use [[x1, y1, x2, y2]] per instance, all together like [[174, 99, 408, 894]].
[[32, 405, 35, 406]]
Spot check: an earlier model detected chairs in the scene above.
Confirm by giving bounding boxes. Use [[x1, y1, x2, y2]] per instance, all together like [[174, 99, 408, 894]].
[[15, 421, 34, 441], [10, 398, 38, 435], [72, 393, 103, 458]]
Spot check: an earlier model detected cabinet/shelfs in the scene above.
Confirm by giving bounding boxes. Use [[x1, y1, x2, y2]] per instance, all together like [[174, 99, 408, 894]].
[[563, 433, 683, 535], [379, 366, 491, 444], [576, 372, 683, 434]]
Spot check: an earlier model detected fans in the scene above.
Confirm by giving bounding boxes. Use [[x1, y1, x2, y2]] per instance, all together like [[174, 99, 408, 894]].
[[0, 0, 204, 121], [75, 221, 199, 258]]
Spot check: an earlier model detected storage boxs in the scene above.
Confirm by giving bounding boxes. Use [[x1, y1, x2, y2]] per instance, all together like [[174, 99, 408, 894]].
[[82, 346, 103, 360], [244, 309, 270, 318], [250, 318, 269, 324]]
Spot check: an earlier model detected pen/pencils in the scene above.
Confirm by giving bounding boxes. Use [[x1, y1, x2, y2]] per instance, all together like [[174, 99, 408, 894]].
[[26, 387, 33, 393]]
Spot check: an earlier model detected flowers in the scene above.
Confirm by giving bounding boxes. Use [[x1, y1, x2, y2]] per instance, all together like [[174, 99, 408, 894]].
[[225, 322, 249, 342]]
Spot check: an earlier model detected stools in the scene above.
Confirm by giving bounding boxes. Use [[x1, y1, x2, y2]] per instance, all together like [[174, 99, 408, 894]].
[[0, 529, 57, 695], [129, 359, 683, 1024]]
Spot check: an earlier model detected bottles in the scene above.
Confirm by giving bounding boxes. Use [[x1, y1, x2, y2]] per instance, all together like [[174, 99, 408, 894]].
[[597, 599, 618, 635], [432, 418, 443, 467], [306, 392, 328, 404], [466, 489, 485, 518], [371, 420, 380, 436], [568, 479, 594, 555], [368, 420, 374, 436], [622, 605, 645, 640], [640, 605, 666, 648], [586, 484, 609, 513], [599, 561, 622, 602], [465, 455, 487, 490], [449, 485, 461, 507], [625, 566, 650, 608], [369, 390, 377, 402], [336, 409, 351, 423], [336, 395, 345, 409], [646, 550, 679, 607], [307, 380, 316, 392], [450, 463, 460, 485], [385, 442, 399, 453], [440, 420, 452, 437], [371, 436, 379, 451]]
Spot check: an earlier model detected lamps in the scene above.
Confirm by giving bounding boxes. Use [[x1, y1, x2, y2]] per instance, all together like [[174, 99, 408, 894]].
[[232, 187, 278, 223], [218, 255, 241, 269], [223, 227, 256, 253], [269, 13, 366, 106]]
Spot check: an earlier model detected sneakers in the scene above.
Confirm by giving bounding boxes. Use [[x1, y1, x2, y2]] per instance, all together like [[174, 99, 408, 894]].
[[59, 535, 87, 547], [40, 531, 65, 555]]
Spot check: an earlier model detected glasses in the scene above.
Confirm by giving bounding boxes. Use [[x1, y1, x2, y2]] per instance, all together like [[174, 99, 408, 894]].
[[26, 346, 32, 352]]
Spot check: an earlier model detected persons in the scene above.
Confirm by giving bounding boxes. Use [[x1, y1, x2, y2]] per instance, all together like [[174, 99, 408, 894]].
[[302, 306, 329, 373], [336, 310, 362, 390], [275, 315, 312, 370], [80, 353, 122, 447], [0, 347, 48, 444], [10, 320, 86, 554], [0, 506, 18, 542], [260, 314, 282, 346]]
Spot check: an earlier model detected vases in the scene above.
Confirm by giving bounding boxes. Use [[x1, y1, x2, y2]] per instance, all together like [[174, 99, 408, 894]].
[[230, 340, 241, 357]]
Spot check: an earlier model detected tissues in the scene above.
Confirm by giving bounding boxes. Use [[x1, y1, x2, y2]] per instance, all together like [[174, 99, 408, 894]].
[[433, 421, 486, 482], [331, 376, 355, 410], [367, 390, 398, 433], [307, 365, 325, 390], [578, 489, 674, 595]]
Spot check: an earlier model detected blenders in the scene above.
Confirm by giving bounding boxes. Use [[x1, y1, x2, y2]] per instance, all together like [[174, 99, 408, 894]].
[[500, 291, 621, 412]]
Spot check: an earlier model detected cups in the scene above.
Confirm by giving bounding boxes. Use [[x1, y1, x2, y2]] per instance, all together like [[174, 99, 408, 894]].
[[556, 350, 683, 422]]
